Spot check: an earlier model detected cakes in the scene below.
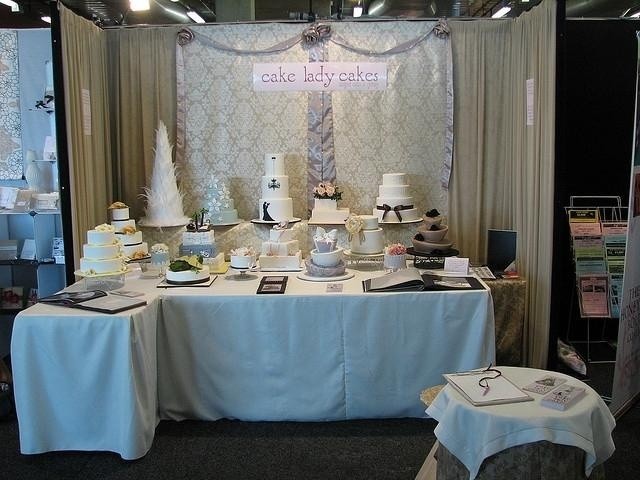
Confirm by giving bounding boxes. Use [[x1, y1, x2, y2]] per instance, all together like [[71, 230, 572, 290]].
[[137, 119, 188, 226], [372, 172, 418, 222], [165, 253, 210, 284], [107, 201, 148, 261], [383, 243, 407, 269], [305, 227, 346, 277], [258, 154, 293, 221], [200, 175, 238, 224], [410, 208, 454, 254], [344, 213, 384, 254], [311, 180, 350, 222], [182, 208, 225, 270], [230, 246, 257, 270], [259, 219, 302, 269], [150, 243, 170, 265], [80, 223, 125, 274]]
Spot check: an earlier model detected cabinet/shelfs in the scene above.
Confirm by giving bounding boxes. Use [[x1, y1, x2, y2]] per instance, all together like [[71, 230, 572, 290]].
[[0, 108, 62, 315]]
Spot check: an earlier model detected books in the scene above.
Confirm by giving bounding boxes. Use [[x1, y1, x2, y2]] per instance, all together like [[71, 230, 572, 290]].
[[36, 290, 147, 315], [362, 269, 485, 291]]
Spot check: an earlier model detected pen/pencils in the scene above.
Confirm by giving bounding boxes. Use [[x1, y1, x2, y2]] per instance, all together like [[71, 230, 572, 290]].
[[481, 387, 489, 396]]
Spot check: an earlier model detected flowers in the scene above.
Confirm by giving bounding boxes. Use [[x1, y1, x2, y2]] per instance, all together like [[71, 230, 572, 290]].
[[312, 181, 342, 200], [151, 243, 169, 254], [230, 246, 260, 257], [385, 243, 407, 255]]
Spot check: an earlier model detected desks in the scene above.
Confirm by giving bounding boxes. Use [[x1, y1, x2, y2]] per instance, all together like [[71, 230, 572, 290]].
[[424, 370, 599, 479], [14, 258, 528, 460]]
